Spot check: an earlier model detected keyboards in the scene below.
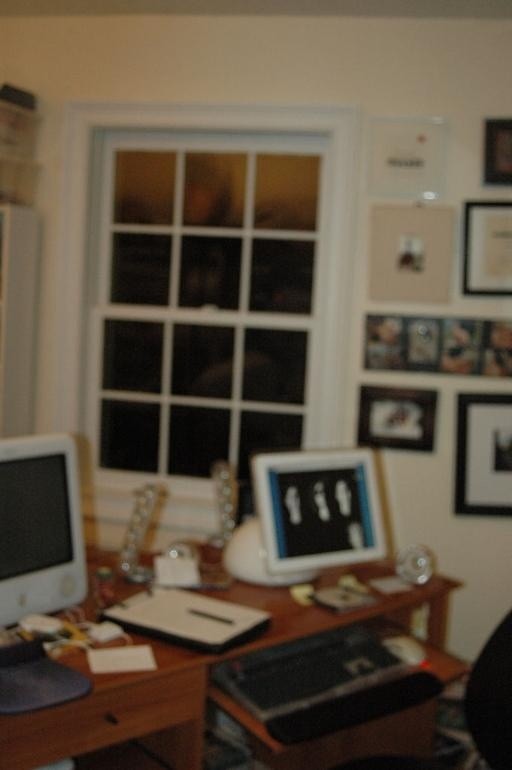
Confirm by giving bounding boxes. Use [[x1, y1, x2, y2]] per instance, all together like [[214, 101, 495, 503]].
[[225, 631, 400, 711]]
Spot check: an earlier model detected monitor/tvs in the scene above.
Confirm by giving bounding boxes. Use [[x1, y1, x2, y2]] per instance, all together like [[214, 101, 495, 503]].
[[246, 445, 392, 571], [0, 430, 90, 630]]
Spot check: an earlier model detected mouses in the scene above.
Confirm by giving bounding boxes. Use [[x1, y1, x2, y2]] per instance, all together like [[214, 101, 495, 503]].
[[383, 635, 428, 665]]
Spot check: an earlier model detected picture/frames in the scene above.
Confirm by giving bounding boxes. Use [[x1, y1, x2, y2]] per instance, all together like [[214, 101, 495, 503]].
[[461, 116, 510, 296]]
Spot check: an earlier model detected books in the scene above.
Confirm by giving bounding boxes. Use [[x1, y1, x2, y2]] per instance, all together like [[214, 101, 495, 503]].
[[101, 586, 274, 655]]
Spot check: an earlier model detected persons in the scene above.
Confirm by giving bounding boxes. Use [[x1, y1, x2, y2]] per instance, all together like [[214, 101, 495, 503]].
[[283, 486, 313, 556], [335, 479, 366, 549], [309, 478, 339, 552]]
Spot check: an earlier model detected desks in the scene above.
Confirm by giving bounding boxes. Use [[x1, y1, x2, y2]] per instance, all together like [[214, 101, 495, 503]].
[[1, 543, 463, 767]]
